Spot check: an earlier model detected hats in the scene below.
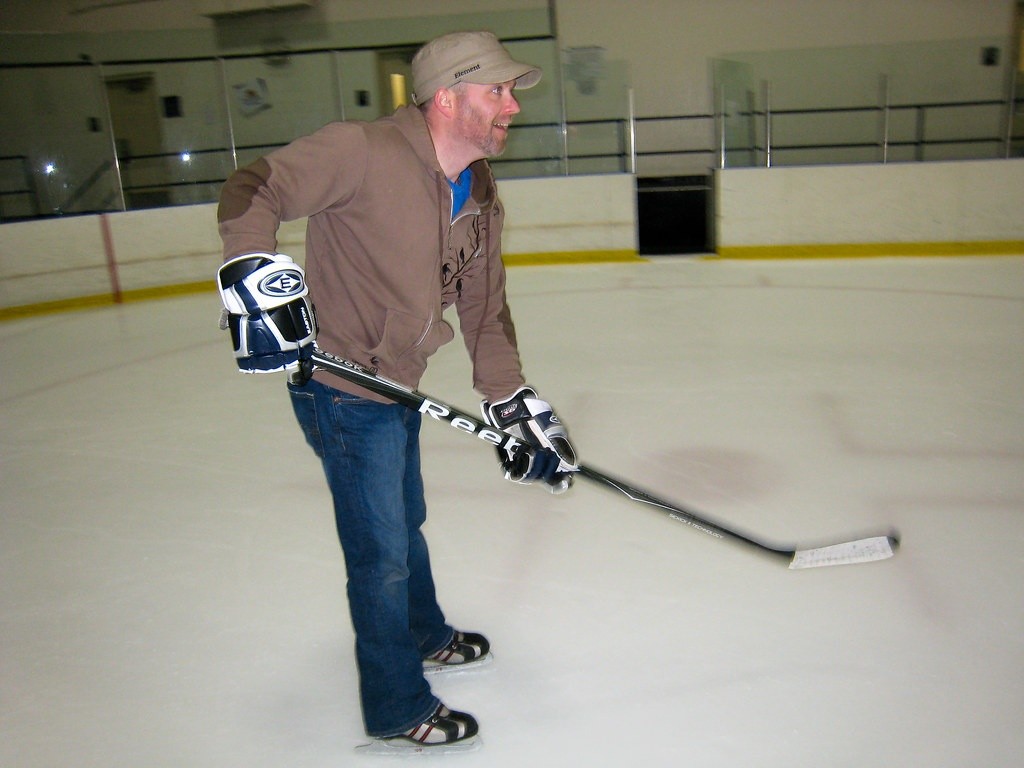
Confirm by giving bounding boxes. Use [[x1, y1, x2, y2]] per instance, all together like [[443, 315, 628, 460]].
[[411, 30, 541, 106]]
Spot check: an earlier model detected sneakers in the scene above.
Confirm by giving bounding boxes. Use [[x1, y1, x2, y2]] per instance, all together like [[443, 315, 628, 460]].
[[355, 703, 483, 756], [420, 631, 494, 675]]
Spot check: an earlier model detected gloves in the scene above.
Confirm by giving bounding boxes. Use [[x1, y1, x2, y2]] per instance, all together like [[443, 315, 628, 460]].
[[217, 252, 321, 385], [480, 386, 578, 494]]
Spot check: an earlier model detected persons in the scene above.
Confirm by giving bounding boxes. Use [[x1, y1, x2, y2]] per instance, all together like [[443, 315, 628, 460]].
[[216, 30, 576, 749]]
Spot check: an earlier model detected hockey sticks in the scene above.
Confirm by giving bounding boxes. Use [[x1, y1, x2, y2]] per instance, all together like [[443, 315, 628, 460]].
[[215, 305, 903, 573]]
